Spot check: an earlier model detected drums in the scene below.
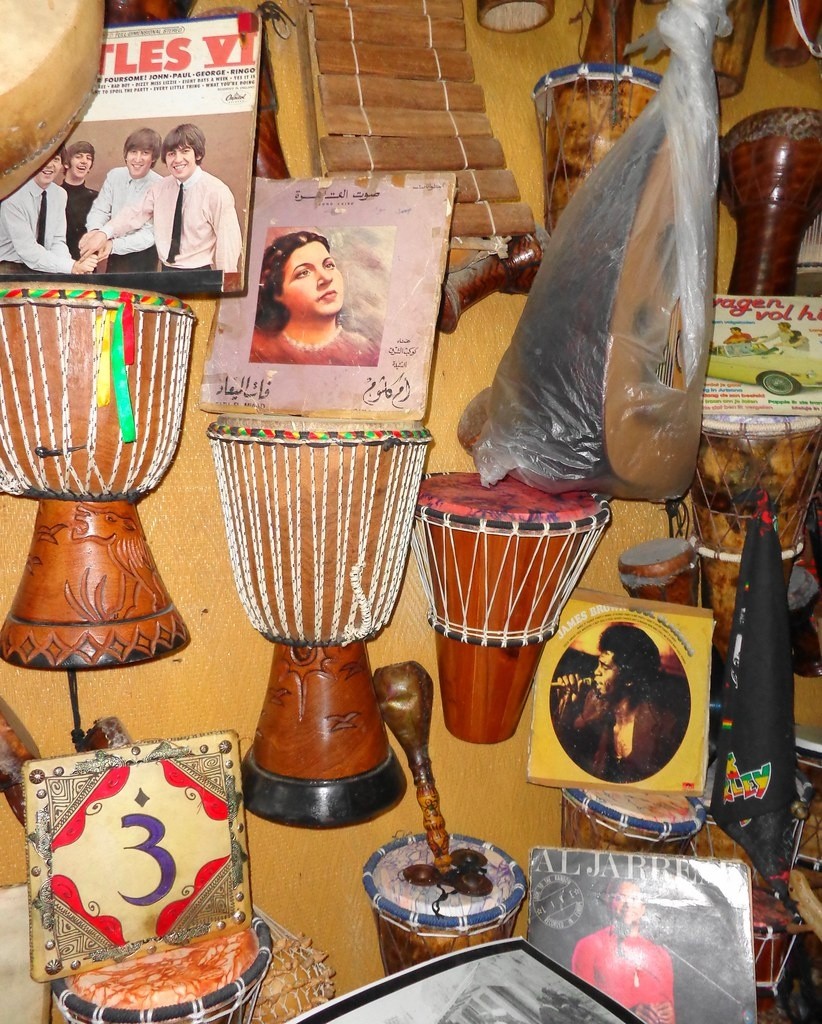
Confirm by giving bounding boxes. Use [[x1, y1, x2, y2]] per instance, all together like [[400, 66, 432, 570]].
[[561, 790, 706, 854], [691, 415, 821, 663], [620, 538, 703, 606], [210, 410, 435, 831], [408, 471, 614, 745], [50, 910, 270, 1024], [752, 887, 800, 1008], [477, 1, 821, 98], [0, 1, 105, 201], [716, 106, 822, 295], [364, 831, 525, 976], [1, 283, 195, 670], [532, 63, 663, 237], [436, 231, 540, 334]]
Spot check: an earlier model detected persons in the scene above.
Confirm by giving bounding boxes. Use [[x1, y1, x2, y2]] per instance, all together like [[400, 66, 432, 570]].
[[571, 876, 676, 1024], [78, 123, 242, 273], [60, 141, 100, 273], [551, 624, 684, 784], [722, 319, 810, 351], [0, 142, 99, 276], [249, 231, 380, 367], [76, 127, 162, 274]]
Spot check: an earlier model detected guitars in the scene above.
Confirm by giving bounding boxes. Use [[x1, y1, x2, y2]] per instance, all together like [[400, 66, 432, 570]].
[[460, 2, 741, 503]]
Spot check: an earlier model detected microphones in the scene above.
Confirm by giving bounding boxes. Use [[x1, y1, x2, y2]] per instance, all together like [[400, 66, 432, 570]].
[[551, 677, 594, 689], [639, 916, 655, 940]]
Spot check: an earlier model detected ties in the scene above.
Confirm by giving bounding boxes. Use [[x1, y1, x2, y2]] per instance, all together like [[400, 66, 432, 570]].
[[37, 191, 47, 246], [167, 184, 183, 264]]
